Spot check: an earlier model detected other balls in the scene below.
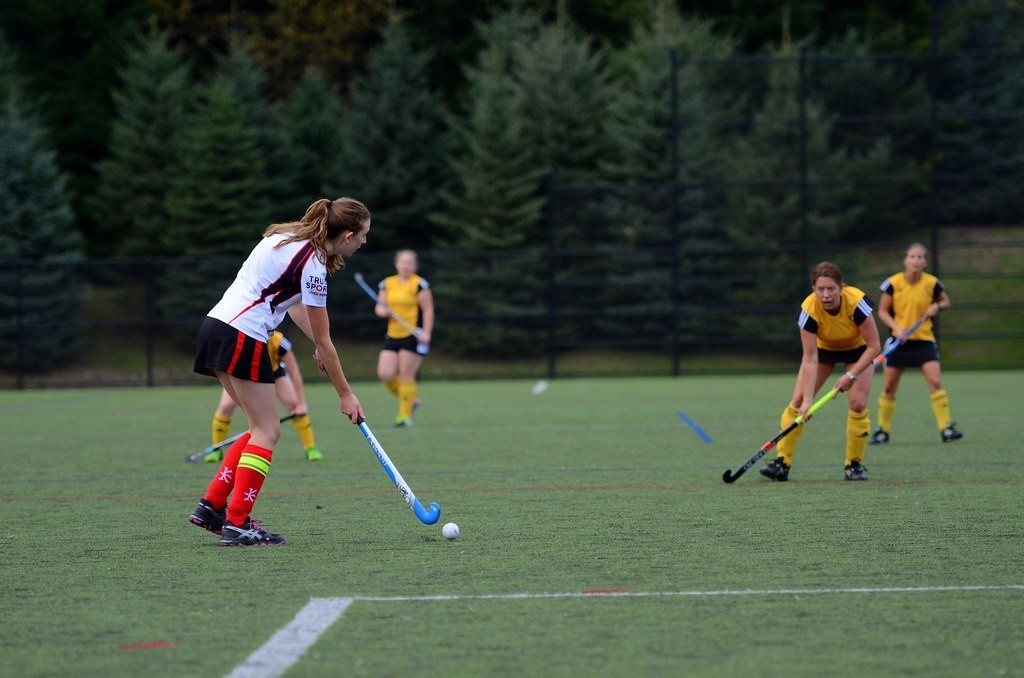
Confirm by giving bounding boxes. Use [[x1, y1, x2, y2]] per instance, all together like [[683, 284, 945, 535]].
[[442, 522, 460, 540]]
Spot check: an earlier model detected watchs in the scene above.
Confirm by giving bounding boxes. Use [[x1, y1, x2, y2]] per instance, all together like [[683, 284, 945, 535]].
[[847, 371, 857, 381]]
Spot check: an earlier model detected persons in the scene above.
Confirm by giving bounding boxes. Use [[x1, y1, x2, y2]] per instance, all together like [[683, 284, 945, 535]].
[[375, 250, 434, 426], [760, 263, 881, 482], [189, 197, 371, 545], [205, 331, 321, 462], [870, 245, 963, 443]]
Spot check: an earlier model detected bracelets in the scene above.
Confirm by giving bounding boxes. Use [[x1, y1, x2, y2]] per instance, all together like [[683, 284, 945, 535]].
[[936, 303, 943, 311]]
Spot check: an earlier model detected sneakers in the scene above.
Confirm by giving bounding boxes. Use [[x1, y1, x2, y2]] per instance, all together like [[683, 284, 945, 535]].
[[204, 449, 224, 462], [941, 422, 963, 443], [760, 457, 791, 481], [868, 426, 889, 445], [412, 397, 421, 413], [844, 459, 868, 480], [305, 447, 323, 461], [395, 414, 412, 426], [188, 498, 228, 535], [219, 516, 286, 548]]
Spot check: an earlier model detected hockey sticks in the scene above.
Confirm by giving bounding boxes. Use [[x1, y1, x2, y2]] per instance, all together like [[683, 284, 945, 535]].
[[187, 414, 296, 462], [723, 387, 838, 483], [871, 319, 920, 365], [312, 352, 441, 525], [354, 272, 421, 339]]
